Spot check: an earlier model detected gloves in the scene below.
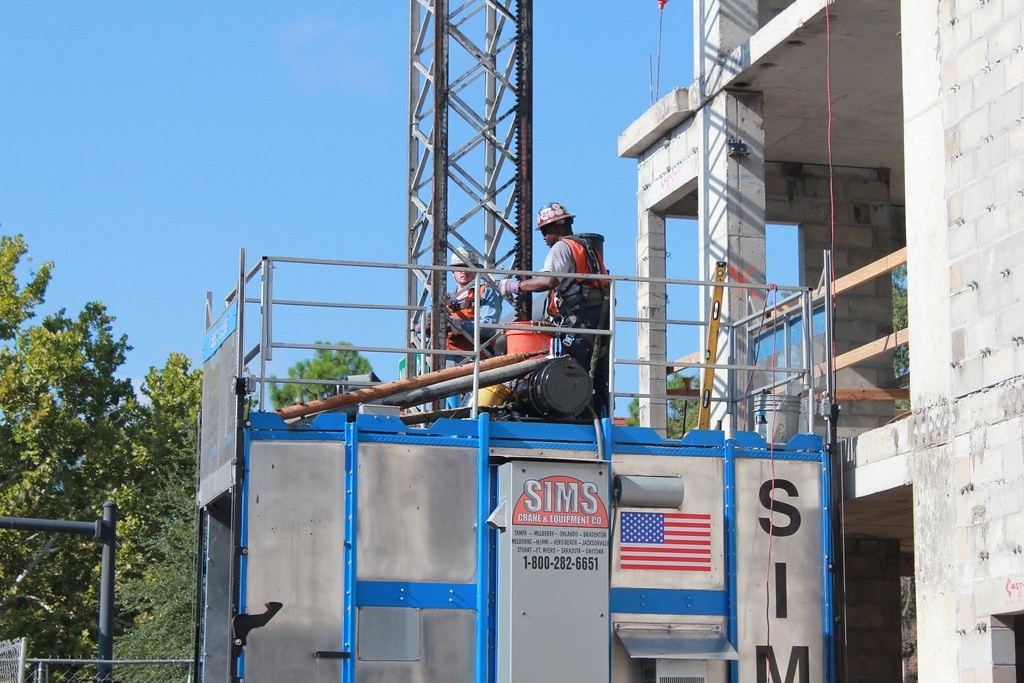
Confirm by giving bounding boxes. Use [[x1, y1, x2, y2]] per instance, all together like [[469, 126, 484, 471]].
[[500, 279, 522, 295]]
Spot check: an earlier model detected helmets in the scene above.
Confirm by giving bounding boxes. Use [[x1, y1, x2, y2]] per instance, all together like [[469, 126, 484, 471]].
[[534, 201, 576, 230], [449, 246, 485, 267]]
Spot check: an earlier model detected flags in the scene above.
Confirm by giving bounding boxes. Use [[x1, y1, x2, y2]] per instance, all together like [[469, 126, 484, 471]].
[[620, 511, 712, 572]]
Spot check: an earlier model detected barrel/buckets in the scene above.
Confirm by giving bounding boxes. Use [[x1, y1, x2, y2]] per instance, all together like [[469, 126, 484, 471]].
[[476, 383, 512, 420], [501, 320, 552, 371], [753, 379, 801, 444]]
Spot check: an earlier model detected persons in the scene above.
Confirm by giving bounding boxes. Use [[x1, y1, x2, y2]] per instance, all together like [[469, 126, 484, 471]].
[[498, 202, 613, 426], [414, 246, 502, 408]]
